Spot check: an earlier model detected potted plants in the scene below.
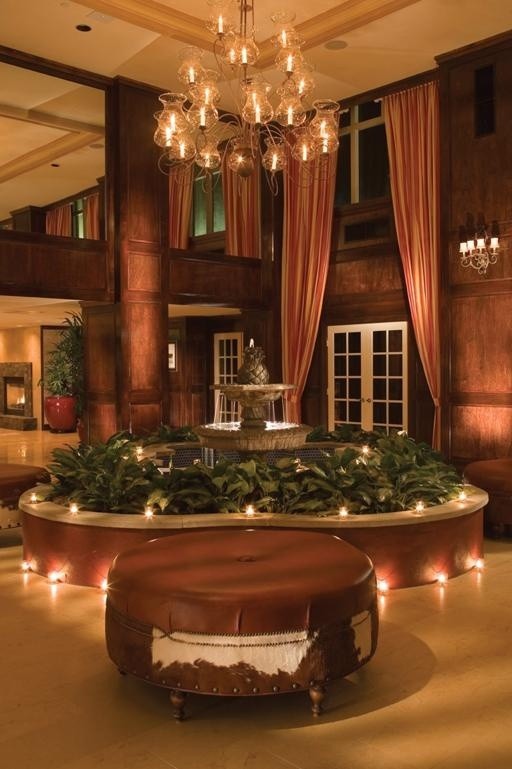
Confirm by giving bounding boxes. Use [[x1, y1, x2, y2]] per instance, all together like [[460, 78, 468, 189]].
[[36, 310, 83, 441]]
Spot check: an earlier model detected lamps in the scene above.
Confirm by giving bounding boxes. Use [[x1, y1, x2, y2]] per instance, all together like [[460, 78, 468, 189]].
[[459, 237, 500, 275], [153, 0, 342, 196]]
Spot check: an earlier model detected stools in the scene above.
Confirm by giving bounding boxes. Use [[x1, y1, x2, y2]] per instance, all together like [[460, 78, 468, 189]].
[[105, 529, 378, 724], [0, 463, 51, 530], [463, 458, 512, 540]]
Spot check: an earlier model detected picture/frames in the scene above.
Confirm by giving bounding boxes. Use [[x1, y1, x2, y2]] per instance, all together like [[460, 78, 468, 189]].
[[168, 340, 178, 373]]
